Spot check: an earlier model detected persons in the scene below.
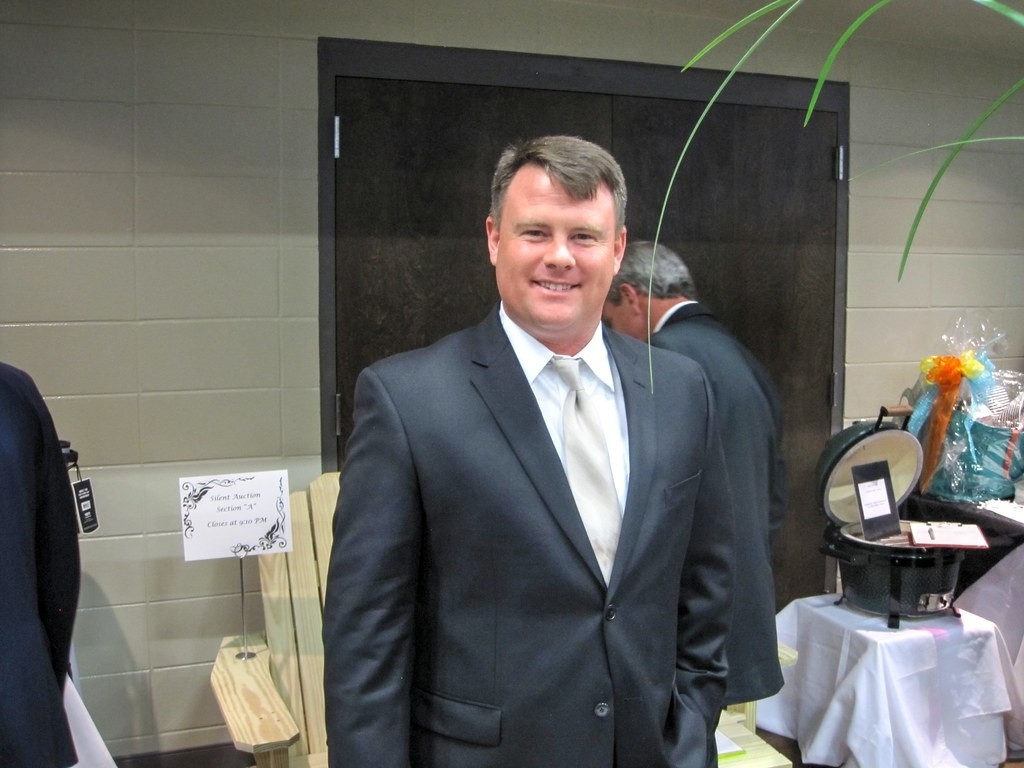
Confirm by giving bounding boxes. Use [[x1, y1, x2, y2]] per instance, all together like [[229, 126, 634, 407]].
[[604, 237, 791, 767], [0, 360, 79, 768], [323, 137, 738, 767]]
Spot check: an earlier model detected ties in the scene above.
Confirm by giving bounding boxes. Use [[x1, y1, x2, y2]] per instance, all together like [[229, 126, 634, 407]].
[[550, 356, 623, 587]]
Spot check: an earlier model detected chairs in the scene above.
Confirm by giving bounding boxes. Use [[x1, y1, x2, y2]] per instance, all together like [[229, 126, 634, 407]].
[[208, 472, 801, 768]]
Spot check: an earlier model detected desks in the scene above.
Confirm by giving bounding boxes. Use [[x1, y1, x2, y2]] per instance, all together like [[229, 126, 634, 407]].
[[899, 481, 1024, 760], [794, 593, 998, 768]]
